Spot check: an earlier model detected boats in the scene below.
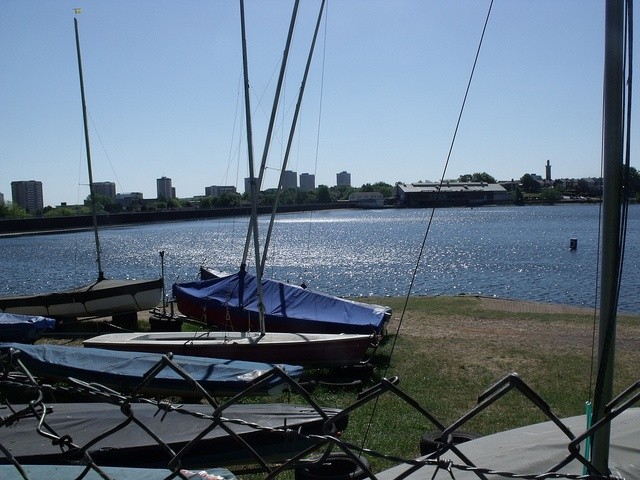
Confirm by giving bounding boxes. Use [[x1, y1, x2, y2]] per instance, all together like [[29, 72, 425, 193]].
[[0, 312, 56, 344], [82, 332, 382, 369], [173, 270, 389, 333], [0, 341, 305, 400], [200, 265, 231, 281], [1, 402, 349, 467], [0, 276, 162, 321]]
